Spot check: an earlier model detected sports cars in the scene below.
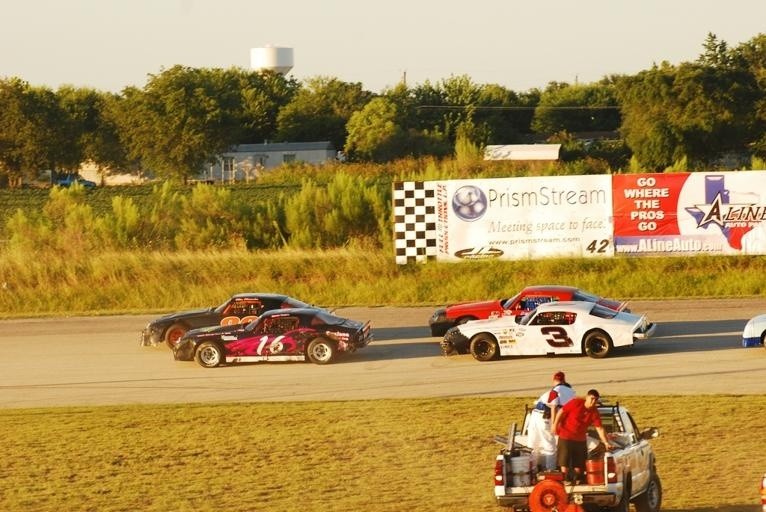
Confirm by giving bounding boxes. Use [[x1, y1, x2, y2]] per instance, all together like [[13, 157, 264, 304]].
[[428, 286, 657, 361], [742, 314, 766, 348], [142, 293, 375, 368]]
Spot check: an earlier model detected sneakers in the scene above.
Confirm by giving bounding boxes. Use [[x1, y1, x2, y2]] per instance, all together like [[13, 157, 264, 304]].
[[529, 469, 586, 486]]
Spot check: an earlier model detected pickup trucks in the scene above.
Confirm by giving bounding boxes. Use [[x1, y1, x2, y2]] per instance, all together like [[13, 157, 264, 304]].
[[494, 399, 662, 512]]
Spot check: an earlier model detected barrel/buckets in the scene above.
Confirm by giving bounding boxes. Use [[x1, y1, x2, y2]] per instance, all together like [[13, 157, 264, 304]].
[[511, 456, 530, 486], [584, 459, 603, 485]]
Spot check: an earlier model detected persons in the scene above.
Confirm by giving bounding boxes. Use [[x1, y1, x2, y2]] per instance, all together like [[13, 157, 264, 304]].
[[545, 371, 578, 473], [552, 389, 617, 486]]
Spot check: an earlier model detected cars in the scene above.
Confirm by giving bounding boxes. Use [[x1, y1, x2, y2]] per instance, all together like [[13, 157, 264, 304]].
[[187, 174, 215, 185], [52, 173, 96, 186]]
